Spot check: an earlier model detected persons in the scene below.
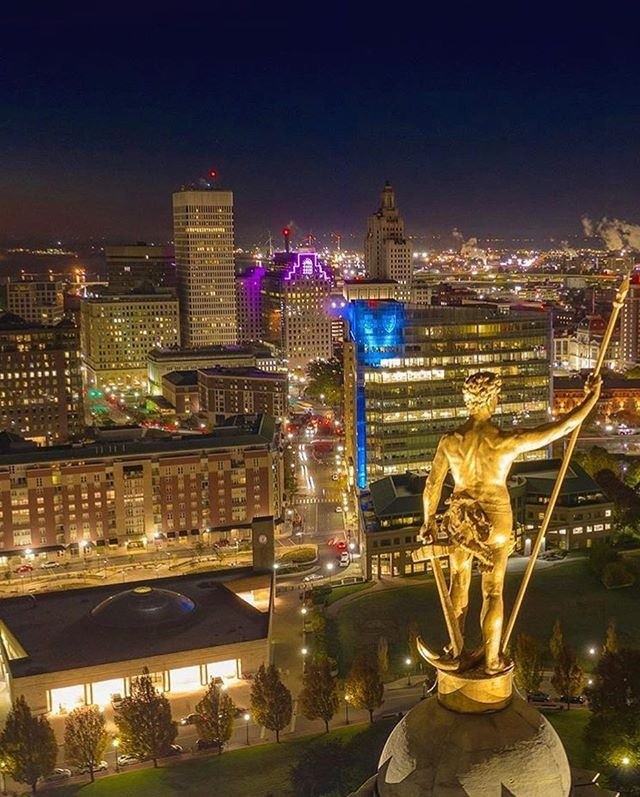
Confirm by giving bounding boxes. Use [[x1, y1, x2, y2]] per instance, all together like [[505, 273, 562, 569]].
[[420, 372, 603, 675]]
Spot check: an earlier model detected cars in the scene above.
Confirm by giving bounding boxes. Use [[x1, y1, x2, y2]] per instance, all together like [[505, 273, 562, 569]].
[[213, 538, 229, 549], [327, 534, 349, 568], [196, 737, 223, 750], [180, 713, 207, 725], [331, 471, 338, 481], [168, 744, 184, 755], [39, 767, 71, 784], [559, 694, 586, 704], [334, 506, 343, 513], [117, 752, 144, 766], [40, 560, 60, 569], [12, 565, 34, 573], [78, 760, 109, 774]]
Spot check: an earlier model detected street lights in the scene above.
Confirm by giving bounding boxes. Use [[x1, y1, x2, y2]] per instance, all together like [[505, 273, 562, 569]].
[[244, 713, 250, 745], [113, 738, 120, 773], [405, 658, 412, 686], [344, 694, 350, 725]]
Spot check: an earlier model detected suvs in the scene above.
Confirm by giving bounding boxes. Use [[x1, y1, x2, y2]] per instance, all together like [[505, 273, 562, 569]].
[[525, 690, 551, 703]]
